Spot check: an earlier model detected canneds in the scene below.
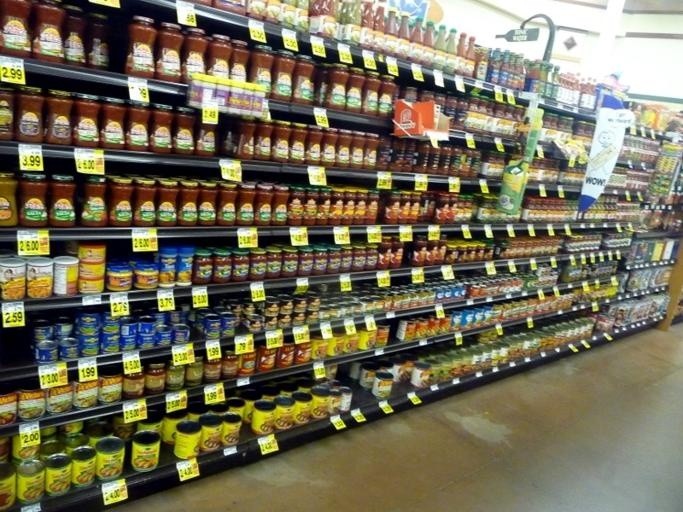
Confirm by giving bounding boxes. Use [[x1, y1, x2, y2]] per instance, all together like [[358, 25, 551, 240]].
[[106, 175, 133, 226], [348, 360, 395, 397], [125, 99, 151, 151], [263, 288, 321, 331], [321, 127, 338, 166], [155, 21, 185, 82], [72, 377, 98, 408], [0, 417, 125, 512], [222, 355, 239, 379], [254, 118, 275, 160], [181, 26, 209, 83], [124, 15, 159, 78], [153, 178, 178, 226], [292, 53, 316, 105], [289, 122, 308, 163], [256, 183, 273, 226], [0, 172, 19, 227], [72, 92, 102, 147], [120, 303, 192, 351], [17, 387, 46, 422], [288, 187, 304, 226], [316, 61, 397, 118], [363, 132, 380, 169], [351, 131, 367, 168], [329, 187, 345, 225], [408, 191, 422, 224], [0, 241, 159, 300], [230, 39, 251, 82], [32, 0, 66, 63], [538, 110, 596, 146], [166, 360, 186, 391], [236, 183, 256, 226], [447, 91, 470, 130], [398, 84, 447, 114], [272, 184, 290, 225], [46, 382, 74, 415], [173, 106, 197, 155], [193, 179, 217, 226], [470, 92, 529, 139], [159, 242, 283, 289], [126, 389, 244, 474], [312, 324, 391, 360], [235, 116, 256, 159], [215, 179, 238, 227], [303, 187, 319, 226], [272, 49, 296, 101], [276, 342, 295, 368], [192, 297, 262, 341], [271, 120, 293, 162], [130, 177, 157, 226], [150, 102, 174, 153], [395, 342, 455, 389], [185, 356, 205, 387], [244, 372, 342, 437], [540, 317, 595, 348], [338, 386, 353, 412], [29, 311, 120, 364], [171, 178, 199, 226], [45, 89, 74, 145], [17, 172, 49, 226], [204, 36, 214, 41], [398, 314, 451, 342], [0, 86, 15, 140], [238, 349, 257, 377], [294, 342, 312, 364], [335, 129, 353, 167], [123, 365, 145, 399], [249, 43, 272, 98], [145, 363, 166, 394], [318, 187, 332, 225], [80, 176, 109, 227], [0, 0, 32, 57], [16, 86, 45, 143], [344, 187, 357, 225], [195, 110, 219, 156], [352, 188, 368, 224], [272, 50, 278, 55], [61, 4, 86, 66], [203, 358, 222, 381], [436, 270, 539, 304], [385, 188, 402, 224], [380, 132, 480, 178], [411, 233, 559, 267], [396, 190, 413, 224], [47, 174, 76, 227], [99, 96, 126, 150], [98, 372, 123, 405], [306, 125, 323, 164], [366, 189, 380, 224], [455, 330, 540, 378], [283, 235, 404, 278], [423, 190, 578, 221], [321, 277, 436, 321], [256, 345, 277, 372], [87, 12, 110, 70], [207, 33, 234, 79], [480, 149, 583, 186], [0, 391, 18, 427]]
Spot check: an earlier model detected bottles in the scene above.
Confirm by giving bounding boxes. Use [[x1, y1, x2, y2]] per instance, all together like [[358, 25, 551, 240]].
[[249, 0, 526, 90], [527, 58, 670, 129]]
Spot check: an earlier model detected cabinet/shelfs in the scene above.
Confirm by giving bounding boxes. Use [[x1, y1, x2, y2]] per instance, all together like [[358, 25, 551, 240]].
[[0, 0, 683, 511]]
